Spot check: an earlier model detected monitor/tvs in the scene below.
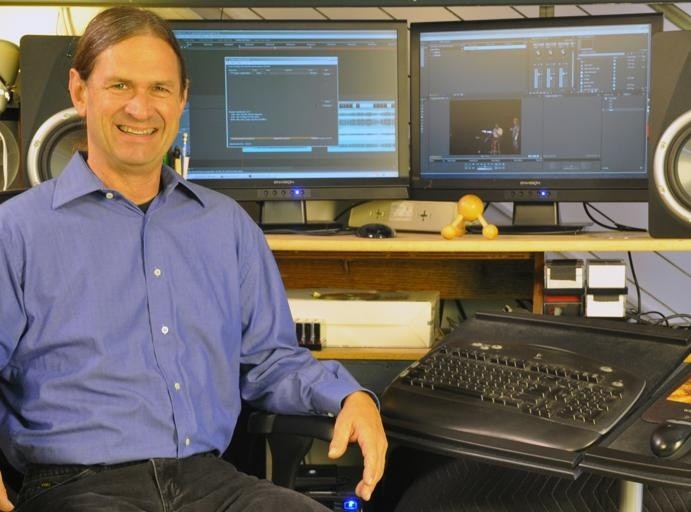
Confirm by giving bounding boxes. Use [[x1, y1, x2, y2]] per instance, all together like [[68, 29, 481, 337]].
[[408, 9, 664, 236], [164, 18, 409, 233]]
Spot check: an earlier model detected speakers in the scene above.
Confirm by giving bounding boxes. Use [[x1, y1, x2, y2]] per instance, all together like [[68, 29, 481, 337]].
[[19, 34, 88, 192], [647, 29, 691, 240]]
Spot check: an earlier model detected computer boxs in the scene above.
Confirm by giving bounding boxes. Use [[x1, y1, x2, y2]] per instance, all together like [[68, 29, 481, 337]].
[[291, 465, 365, 511]]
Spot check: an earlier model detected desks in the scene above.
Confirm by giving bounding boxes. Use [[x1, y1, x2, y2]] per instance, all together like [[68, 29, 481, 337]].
[[264, 234, 691, 359]]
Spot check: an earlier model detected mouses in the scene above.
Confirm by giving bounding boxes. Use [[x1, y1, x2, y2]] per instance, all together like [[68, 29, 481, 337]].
[[356, 221, 396, 239], [649, 417, 691, 460]]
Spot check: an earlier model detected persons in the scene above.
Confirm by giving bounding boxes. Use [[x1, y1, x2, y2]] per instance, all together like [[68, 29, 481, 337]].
[[0, 6, 392, 512], [489, 123, 504, 154], [511, 118, 520, 154]]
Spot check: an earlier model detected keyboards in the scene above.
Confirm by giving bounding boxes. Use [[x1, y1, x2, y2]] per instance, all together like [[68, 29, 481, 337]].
[[379, 337, 647, 452]]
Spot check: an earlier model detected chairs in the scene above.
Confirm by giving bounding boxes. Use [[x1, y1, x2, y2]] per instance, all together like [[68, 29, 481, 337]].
[[216, 412, 367, 512]]
